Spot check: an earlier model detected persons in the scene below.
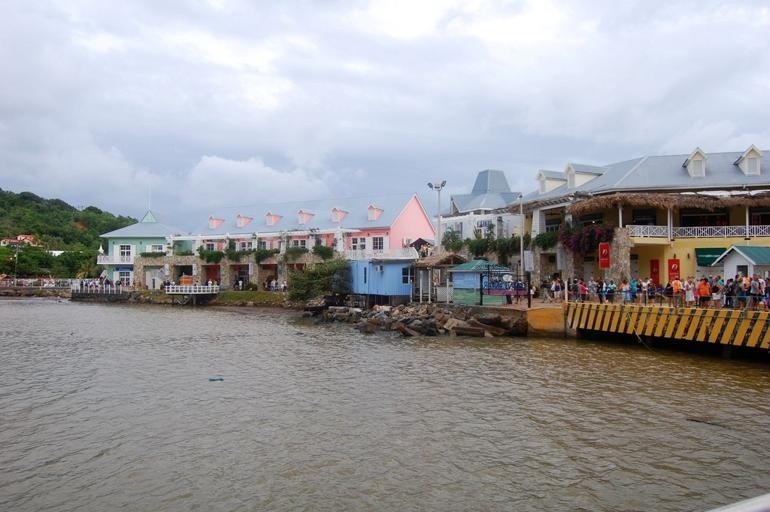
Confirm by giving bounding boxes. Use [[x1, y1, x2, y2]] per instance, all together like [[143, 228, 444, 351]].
[[235, 280, 239, 291], [79, 278, 123, 290], [193, 280, 198, 286], [170, 279, 176, 285], [208, 279, 213, 285], [238, 279, 243, 290], [264, 278, 288, 291], [213, 279, 217, 285], [508, 270, 770, 309], [167, 279, 170, 291]]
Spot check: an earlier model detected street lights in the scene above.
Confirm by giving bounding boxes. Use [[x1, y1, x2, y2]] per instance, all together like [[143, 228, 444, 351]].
[[516, 191, 525, 283], [4, 236, 27, 285], [427, 179, 446, 255]]
